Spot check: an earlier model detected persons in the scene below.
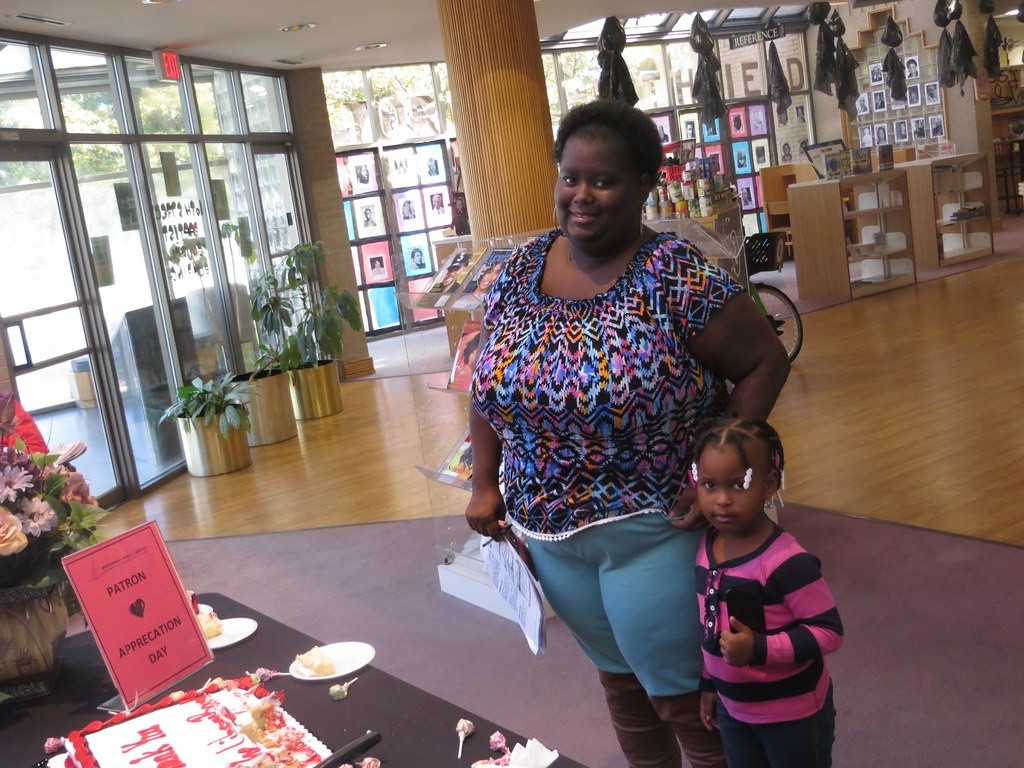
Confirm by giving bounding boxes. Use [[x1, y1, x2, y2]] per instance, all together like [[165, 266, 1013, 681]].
[[357, 159, 507, 391], [690, 415, 845, 768], [466, 99, 791, 768], [854, 59, 945, 148]]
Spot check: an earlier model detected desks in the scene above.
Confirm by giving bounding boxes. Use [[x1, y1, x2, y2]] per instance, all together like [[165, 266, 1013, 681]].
[[1, 591, 590, 766]]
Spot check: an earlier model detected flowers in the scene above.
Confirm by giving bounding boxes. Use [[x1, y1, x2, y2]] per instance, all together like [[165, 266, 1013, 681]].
[[0, 392, 111, 599]]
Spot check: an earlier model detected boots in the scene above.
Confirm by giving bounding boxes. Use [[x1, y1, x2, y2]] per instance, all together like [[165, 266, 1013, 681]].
[[649, 686, 727, 768], [598, 669, 683, 768]]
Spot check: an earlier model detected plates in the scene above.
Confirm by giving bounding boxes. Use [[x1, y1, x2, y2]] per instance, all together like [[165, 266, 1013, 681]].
[[289, 641, 376, 680], [205, 617, 258, 650]]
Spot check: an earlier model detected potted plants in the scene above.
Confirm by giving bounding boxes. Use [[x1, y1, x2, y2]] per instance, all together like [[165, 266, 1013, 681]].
[[158, 372, 259, 478], [229, 267, 305, 446], [284, 240, 362, 420]]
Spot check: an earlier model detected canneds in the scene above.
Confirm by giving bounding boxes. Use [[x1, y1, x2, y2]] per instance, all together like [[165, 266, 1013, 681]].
[[645, 170, 713, 220]]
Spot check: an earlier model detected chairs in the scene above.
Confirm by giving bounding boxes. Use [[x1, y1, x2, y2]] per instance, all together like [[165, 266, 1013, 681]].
[[996, 138, 1024, 216]]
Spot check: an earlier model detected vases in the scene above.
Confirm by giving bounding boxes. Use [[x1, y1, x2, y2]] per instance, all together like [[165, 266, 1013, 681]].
[[0, 581, 68, 698]]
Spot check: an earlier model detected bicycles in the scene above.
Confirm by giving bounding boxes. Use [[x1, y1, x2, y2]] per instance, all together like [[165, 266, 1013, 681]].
[[745, 231, 804, 364]]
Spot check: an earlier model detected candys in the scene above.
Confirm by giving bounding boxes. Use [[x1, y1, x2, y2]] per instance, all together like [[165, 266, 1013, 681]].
[[456, 718, 513, 768], [330, 684, 347, 701], [361, 757, 381, 768], [249, 667, 274, 684], [44, 737, 63, 754]]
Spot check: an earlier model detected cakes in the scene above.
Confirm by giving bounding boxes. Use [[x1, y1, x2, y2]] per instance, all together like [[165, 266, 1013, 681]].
[[197, 612, 223, 638], [294, 645, 337, 678], [63, 678, 290, 768]]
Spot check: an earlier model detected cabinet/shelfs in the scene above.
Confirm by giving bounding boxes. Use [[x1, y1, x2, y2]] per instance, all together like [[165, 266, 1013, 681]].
[[650, 203, 750, 301], [760, 152, 1004, 300], [989, 64, 1024, 155]]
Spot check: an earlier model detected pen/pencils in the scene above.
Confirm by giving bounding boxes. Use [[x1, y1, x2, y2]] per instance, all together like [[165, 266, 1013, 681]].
[[482, 523, 513, 547], [313, 729, 381, 768]]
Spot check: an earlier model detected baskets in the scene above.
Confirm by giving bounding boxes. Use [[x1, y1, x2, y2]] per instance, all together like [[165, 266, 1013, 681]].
[[743, 231, 785, 272]]
